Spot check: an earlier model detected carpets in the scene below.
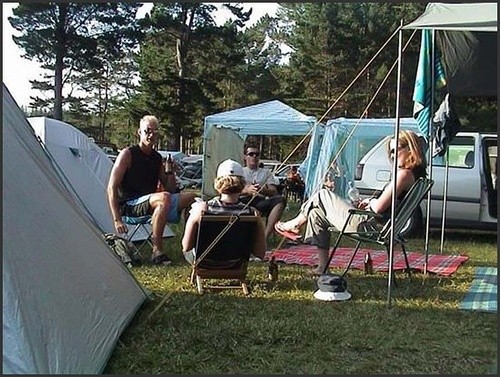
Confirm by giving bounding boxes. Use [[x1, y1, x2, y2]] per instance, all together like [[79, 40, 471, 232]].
[[263, 244, 469, 277], [456, 266, 497, 314]]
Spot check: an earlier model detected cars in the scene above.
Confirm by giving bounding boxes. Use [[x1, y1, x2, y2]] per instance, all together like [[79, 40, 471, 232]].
[[158, 150, 303, 184]]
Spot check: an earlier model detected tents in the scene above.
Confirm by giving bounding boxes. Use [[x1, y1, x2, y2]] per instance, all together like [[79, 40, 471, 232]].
[[0, 81, 149, 377], [26, 117, 176, 241], [156, 100, 325, 201], [401, 0, 500, 256], [312, 118, 444, 199]]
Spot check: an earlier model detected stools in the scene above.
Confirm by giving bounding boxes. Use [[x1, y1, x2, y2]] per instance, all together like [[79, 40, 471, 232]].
[[122, 215, 154, 259]]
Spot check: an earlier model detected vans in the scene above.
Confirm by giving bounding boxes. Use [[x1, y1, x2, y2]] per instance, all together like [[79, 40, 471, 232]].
[[353, 131, 497, 238]]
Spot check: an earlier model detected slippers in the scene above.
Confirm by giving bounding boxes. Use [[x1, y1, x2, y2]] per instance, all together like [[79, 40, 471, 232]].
[[275, 221, 303, 241]]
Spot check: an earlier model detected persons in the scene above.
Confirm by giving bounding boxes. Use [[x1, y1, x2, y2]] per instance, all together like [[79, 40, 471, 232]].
[[106, 115, 198, 266], [182, 159, 266, 271], [287, 166, 306, 199], [274, 131, 427, 275], [239, 145, 287, 241]]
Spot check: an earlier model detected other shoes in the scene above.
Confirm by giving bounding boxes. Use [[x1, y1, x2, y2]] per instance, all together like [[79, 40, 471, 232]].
[[153, 254, 171, 265]]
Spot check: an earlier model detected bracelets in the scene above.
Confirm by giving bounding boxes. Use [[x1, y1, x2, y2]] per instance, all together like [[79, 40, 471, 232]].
[[369, 196, 376, 203], [111, 217, 121, 223]]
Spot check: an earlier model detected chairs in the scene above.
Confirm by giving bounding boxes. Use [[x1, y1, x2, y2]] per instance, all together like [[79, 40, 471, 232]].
[[323, 177, 435, 289], [191, 210, 259, 295], [465, 151, 474, 167], [284, 177, 304, 203]]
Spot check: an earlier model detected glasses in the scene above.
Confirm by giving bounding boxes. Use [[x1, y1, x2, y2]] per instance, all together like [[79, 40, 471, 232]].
[[391, 144, 407, 154], [247, 152, 260, 156], [144, 128, 159, 135]]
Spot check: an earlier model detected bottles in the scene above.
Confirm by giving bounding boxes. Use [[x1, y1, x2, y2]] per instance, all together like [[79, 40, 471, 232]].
[[364, 252, 373, 276], [252, 181, 260, 202], [165, 153, 173, 174], [268, 256, 279, 284], [348, 181, 362, 208]]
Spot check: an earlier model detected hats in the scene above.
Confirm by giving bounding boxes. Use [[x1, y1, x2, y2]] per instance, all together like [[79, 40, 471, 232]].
[[313, 274, 352, 301], [217, 159, 245, 179]]
